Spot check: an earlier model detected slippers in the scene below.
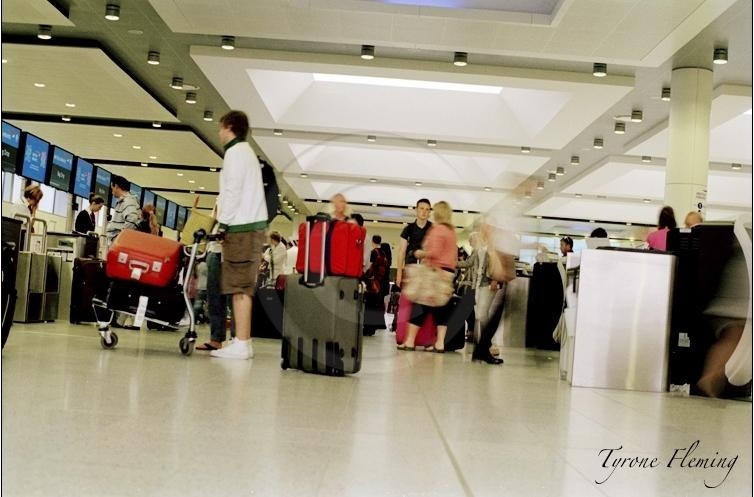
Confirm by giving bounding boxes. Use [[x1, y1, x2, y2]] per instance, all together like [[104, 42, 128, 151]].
[[397, 344, 415, 351], [196, 344, 218, 350], [424, 344, 445, 353]]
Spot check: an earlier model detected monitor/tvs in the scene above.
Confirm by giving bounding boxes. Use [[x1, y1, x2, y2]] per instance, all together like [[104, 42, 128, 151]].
[[164, 199, 178, 230], [129, 182, 143, 210], [72, 156, 95, 200], [46, 144, 75, 194], [1, 120, 22, 174], [110, 174, 119, 208], [186, 209, 192, 220], [156, 194, 168, 226], [93, 166, 112, 207], [176, 204, 187, 231], [17, 131, 51, 185], [142, 189, 156, 214]]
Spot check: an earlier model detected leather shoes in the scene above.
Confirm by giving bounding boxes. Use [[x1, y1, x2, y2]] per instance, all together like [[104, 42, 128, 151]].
[[472, 355, 503, 364]]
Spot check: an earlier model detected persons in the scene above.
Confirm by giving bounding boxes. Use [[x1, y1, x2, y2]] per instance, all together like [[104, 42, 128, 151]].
[[2, 175, 753, 400], [208, 110, 269, 362]]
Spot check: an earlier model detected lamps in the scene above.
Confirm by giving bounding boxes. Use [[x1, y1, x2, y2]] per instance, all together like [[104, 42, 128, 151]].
[[36, 23, 53, 42], [101, 3, 123, 22], [357, 42, 376, 60], [221, 32, 235, 51], [272, 128, 438, 148], [145, 52, 214, 123], [451, 48, 468, 68], [518, 46, 742, 204]]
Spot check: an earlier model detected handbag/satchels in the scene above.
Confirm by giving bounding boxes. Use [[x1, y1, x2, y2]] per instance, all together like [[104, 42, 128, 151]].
[[404, 263, 455, 306], [384, 282, 401, 333], [256, 153, 279, 222], [297, 220, 366, 278]]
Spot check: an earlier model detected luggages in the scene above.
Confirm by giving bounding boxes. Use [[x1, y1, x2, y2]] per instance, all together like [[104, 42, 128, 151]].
[[281, 215, 367, 375], [107, 281, 187, 322], [107, 229, 184, 287]]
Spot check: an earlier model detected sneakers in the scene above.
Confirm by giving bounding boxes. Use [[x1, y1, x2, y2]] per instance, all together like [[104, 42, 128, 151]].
[[210, 340, 251, 360]]
